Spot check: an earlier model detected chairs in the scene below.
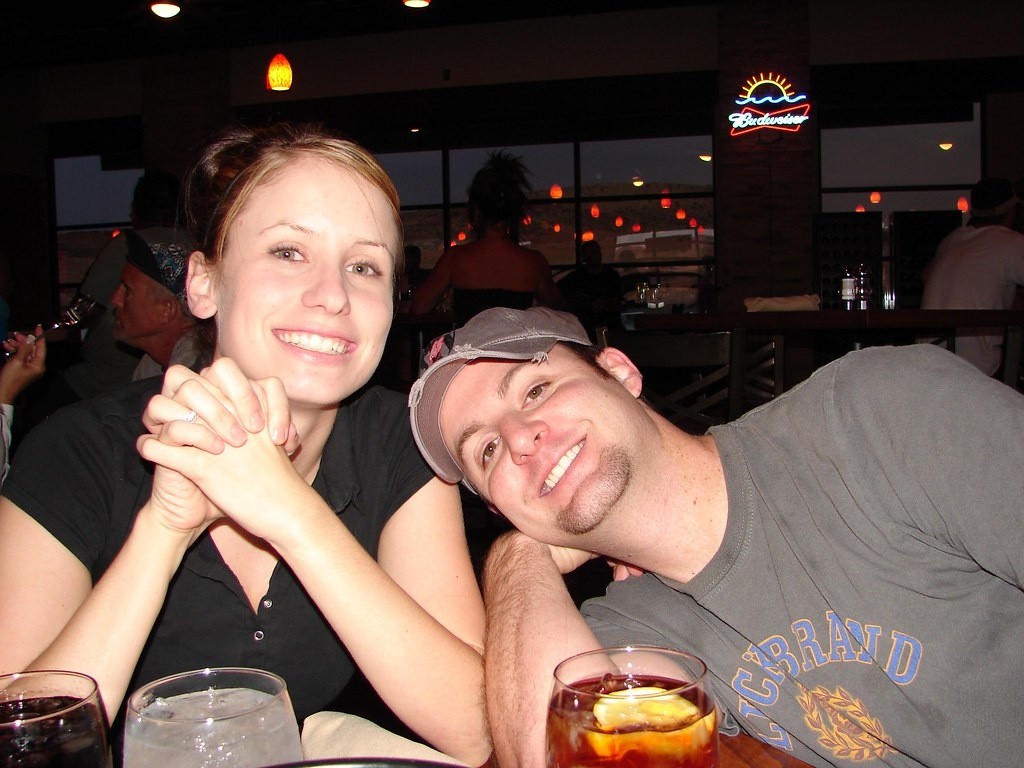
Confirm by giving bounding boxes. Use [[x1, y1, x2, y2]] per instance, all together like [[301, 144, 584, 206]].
[[594, 324, 784, 435]]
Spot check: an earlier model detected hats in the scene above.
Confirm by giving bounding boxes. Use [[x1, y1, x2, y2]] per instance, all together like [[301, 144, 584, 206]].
[[405, 306, 591, 494]]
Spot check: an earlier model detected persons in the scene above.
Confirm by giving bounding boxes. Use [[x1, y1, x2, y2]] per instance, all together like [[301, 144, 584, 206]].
[[0, 120, 1024, 768], [409, 305, 1024, 768]]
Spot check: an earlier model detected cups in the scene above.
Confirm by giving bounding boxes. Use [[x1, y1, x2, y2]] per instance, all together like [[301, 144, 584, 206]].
[[547, 645, 718, 768], [0, 670, 114, 768], [123, 668, 302, 768]]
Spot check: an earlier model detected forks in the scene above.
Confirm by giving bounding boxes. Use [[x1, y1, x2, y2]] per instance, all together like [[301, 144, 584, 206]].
[[4, 294, 96, 361]]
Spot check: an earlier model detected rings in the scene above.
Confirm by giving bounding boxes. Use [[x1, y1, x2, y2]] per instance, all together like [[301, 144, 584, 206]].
[[185, 412, 200, 424]]
